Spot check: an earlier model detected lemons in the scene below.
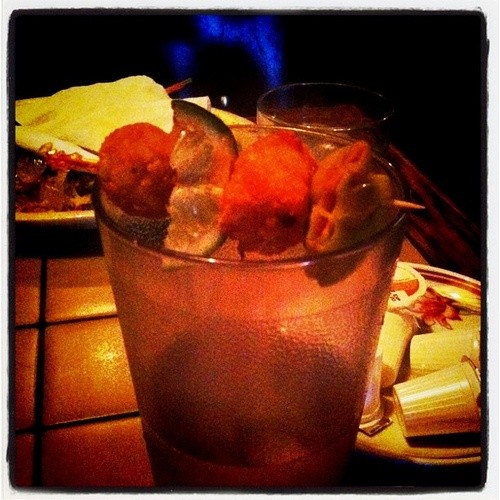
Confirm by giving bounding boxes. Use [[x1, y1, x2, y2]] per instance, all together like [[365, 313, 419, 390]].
[[161, 99, 240, 270]]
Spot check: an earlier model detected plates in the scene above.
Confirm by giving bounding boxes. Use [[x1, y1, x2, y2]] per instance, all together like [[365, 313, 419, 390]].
[[352, 262, 482, 466], [16, 107, 256, 230]]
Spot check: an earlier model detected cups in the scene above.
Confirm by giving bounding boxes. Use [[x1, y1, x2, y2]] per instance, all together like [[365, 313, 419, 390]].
[[257, 82, 393, 164], [90, 121, 412, 493]]
